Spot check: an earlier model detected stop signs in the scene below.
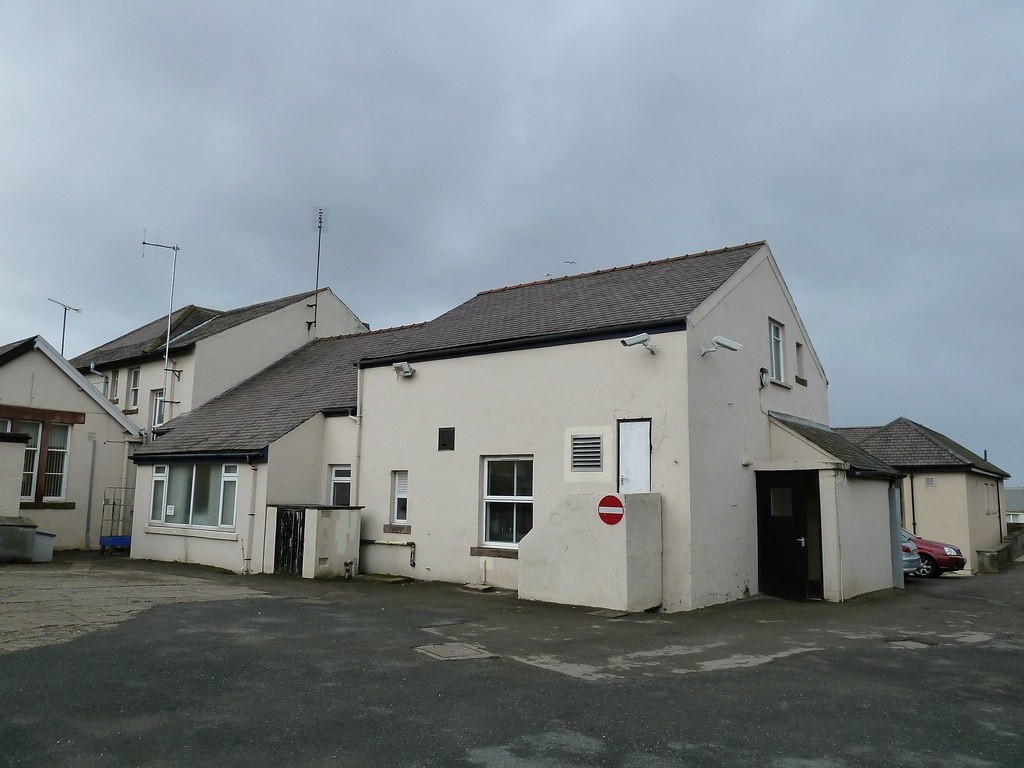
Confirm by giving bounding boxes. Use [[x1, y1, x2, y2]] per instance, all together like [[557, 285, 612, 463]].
[[597, 495, 625, 526]]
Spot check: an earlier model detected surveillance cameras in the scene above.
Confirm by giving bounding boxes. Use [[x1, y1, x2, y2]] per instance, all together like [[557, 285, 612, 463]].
[[621, 333, 650, 347], [713, 336, 744, 352]]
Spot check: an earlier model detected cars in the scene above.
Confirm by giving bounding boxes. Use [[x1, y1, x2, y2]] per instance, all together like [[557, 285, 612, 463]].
[[902, 529, 967, 579], [900, 533, 919, 575]]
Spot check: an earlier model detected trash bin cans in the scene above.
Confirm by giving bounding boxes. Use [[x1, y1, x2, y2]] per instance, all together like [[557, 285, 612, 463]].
[[33, 530, 57, 563]]
